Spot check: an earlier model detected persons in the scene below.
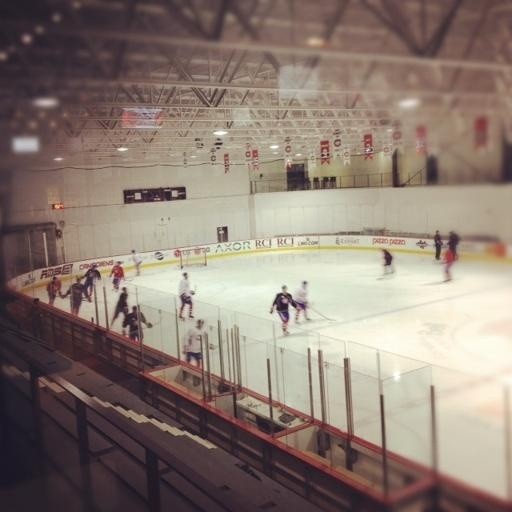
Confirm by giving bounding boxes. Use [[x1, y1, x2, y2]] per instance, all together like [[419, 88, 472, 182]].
[[382, 249, 393, 275], [183, 319, 214, 368], [179, 273, 194, 320], [269, 281, 310, 336], [434, 231, 459, 280], [46, 250, 152, 342]]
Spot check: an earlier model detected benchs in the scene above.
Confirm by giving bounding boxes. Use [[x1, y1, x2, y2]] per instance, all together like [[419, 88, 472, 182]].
[[0, 317, 360, 512]]
